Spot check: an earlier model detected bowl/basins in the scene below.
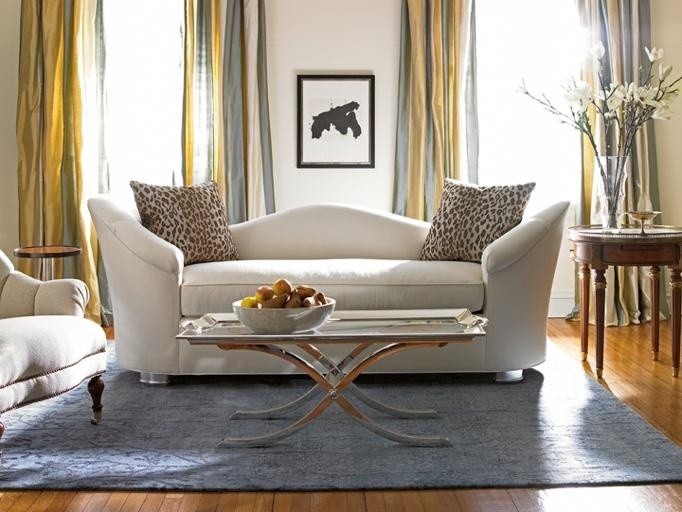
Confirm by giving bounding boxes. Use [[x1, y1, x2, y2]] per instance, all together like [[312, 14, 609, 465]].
[[231, 296, 337, 334]]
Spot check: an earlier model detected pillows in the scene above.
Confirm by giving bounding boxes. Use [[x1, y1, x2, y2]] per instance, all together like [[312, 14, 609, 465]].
[[418, 177, 536, 264], [129, 179, 240, 266]]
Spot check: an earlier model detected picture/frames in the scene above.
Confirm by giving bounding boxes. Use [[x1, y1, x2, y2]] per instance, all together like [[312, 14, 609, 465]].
[[296, 75, 376, 169]]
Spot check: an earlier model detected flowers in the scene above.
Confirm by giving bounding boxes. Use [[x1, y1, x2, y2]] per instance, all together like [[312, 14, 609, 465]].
[[516, 36, 681, 228]]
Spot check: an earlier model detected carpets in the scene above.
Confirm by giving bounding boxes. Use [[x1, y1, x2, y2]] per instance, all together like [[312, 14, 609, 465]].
[[0, 337, 681, 490]]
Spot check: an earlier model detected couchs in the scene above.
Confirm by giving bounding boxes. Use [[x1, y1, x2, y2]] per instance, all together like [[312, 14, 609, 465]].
[[87, 195, 570, 386]]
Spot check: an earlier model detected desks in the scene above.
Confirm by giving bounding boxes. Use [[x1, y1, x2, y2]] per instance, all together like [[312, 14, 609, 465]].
[[567, 224, 682, 378], [13, 245, 81, 280]]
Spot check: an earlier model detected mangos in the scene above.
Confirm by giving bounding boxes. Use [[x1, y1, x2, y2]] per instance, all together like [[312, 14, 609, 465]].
[[241, 278, 327, 308]]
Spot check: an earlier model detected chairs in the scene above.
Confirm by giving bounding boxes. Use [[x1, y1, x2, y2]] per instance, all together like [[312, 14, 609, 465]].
[[1, 247, 108, 458]]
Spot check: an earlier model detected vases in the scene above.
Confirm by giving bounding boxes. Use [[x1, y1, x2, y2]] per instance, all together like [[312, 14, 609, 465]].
[[593, 155, 629, 234]]
